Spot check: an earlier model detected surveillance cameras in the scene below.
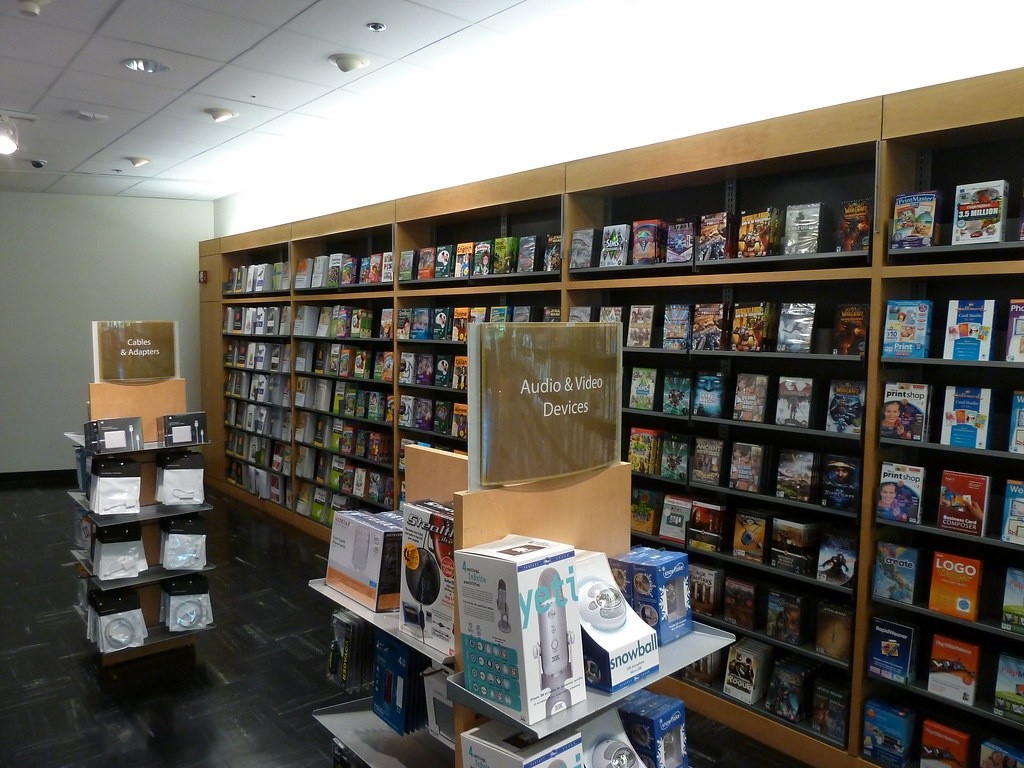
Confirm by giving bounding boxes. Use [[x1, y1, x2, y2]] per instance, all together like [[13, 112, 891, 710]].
[[30, 161, 46, 169]]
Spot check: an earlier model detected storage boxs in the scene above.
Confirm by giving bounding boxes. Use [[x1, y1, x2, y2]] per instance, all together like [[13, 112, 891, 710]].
[[224, 179, 1024, 768]]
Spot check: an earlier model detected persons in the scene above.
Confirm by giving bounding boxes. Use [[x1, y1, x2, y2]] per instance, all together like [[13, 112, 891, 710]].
[[457, 256, 463, 276], [439, 251, 449, 265], [457, 414, 466, 438], [458, 366, 466, 389], [982, 751, 1003, 768], [440, 361, 448, 375], [878, 482, 900, 516], [438, 406, 446, 419], [461, 254, 470, 276], [834, 466, 850, 483], [438, 314, 445, 328], [1003, 755, 1017, 768], [458, 317, 466, 341], [881, 401, 904, 434]]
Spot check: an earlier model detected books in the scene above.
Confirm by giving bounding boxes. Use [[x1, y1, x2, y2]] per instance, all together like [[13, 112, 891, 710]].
[[569, 178, 1024, 768], [225, 233, 562, 529]]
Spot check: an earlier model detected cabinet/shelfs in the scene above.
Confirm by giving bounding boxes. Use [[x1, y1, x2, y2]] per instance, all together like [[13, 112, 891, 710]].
[[563, 94, 883, 768], [198, 222, 293, 523], [853, 67, 1024, 768], [64, 433, 214, 654], [290, 199, 395, 541], [394, 163, 565, 510]]
